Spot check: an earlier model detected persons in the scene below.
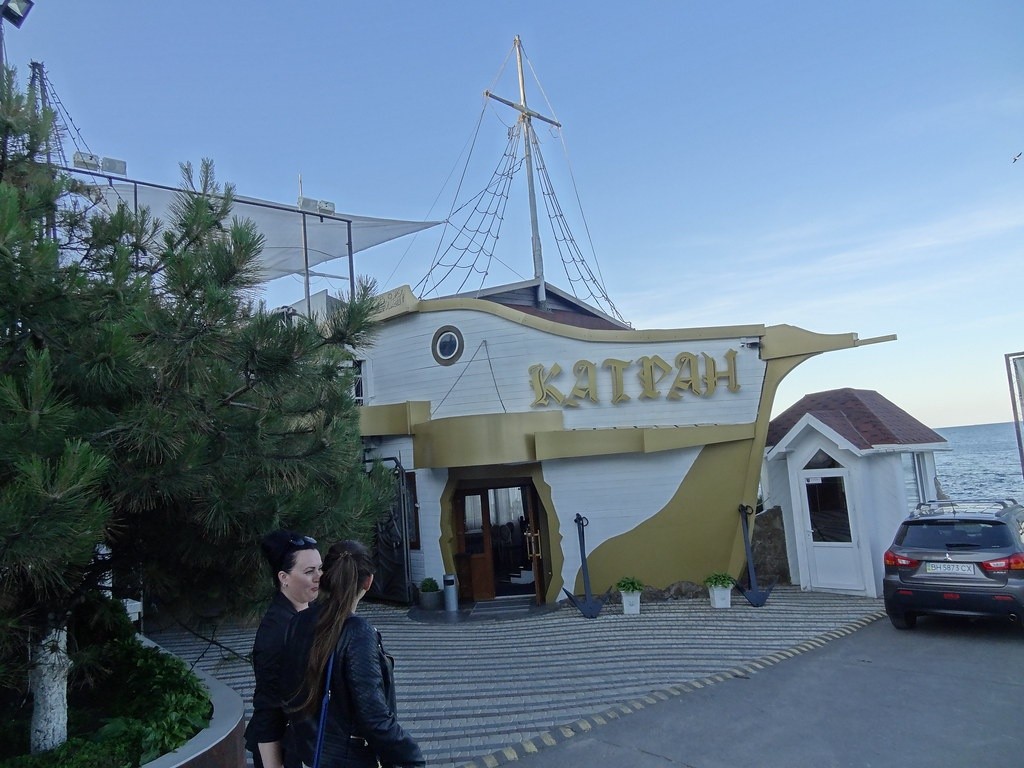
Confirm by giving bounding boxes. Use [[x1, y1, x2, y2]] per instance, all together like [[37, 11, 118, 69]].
[[243, 529, 323, 768], [280, 539, 426, 768]]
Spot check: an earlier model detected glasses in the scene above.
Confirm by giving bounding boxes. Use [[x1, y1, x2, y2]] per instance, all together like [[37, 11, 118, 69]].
[[288, 535, 318, 547]]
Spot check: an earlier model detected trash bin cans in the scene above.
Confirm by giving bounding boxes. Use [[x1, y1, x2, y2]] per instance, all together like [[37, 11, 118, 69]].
[[442, 573, 459, 611]]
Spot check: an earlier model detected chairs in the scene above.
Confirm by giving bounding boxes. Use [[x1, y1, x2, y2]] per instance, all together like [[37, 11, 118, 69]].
[[481, 522, 524, 567]]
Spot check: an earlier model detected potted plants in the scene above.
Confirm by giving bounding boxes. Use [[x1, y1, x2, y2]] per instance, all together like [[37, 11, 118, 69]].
[[617, 576, 645, 615], [703, 571, 736, 609], [417, 577, 442, 611]]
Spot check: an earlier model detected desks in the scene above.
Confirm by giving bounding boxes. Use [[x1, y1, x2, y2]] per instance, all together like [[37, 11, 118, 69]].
[[464, 529, 483, 538]]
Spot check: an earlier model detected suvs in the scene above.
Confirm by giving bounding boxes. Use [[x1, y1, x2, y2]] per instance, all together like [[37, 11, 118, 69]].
[[883, 497, 1023, 632]]
